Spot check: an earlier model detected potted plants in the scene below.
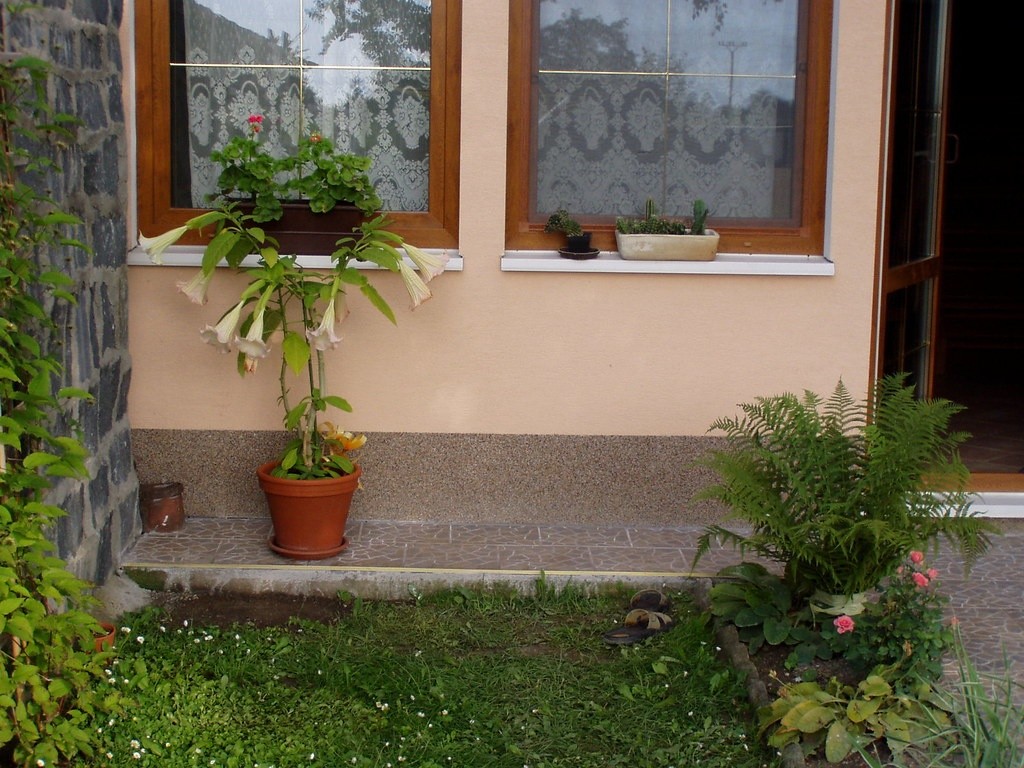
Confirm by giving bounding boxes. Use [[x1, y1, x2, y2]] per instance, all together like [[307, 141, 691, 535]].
[[544, 209, 601, 260], [614, 198, 720, 261]]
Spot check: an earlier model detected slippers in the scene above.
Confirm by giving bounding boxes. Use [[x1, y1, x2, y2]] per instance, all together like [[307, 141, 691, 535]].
[[630, 589, 672, 613], [603, 608, 675, 645]]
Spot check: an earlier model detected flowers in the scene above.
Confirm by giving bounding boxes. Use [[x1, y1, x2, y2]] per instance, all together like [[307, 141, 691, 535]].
[[203, 114, 384, 223], [139, 200, 450, 480]]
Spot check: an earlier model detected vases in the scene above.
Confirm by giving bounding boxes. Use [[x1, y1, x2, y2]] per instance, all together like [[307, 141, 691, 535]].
[[211, 197, 367, 255], [256, 460, 361, 561]]
